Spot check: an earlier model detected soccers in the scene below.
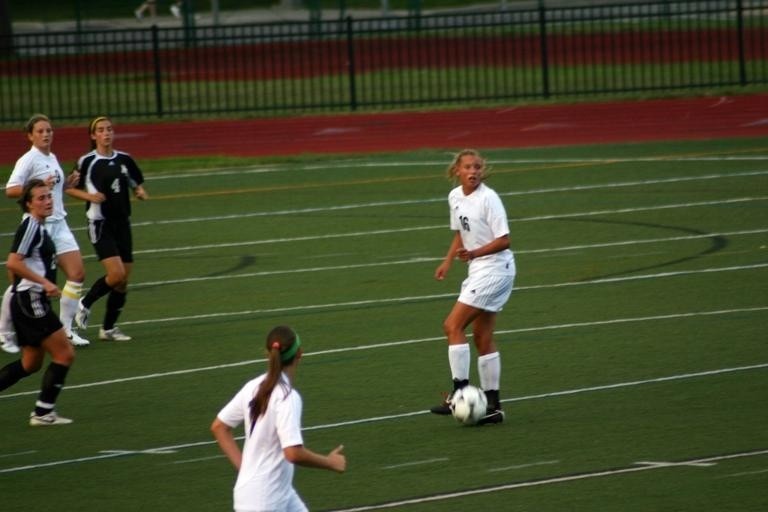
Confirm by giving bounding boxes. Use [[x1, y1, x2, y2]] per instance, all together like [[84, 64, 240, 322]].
[[449, 385, 488, 426]]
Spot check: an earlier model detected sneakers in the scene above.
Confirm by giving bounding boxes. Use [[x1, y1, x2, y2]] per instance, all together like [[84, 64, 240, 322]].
[[74, 296, 90, 329], [474, 401, 504, 426], [65, 333, 90, 347], [429, 393, 456, 416], [0, 335, 20, 354], [98, 325, 133, 341], [28, 411, 74, 427]]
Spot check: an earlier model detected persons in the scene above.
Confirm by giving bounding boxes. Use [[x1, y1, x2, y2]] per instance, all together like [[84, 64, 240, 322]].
[[427, 149, 516, 424], [62, 116, 151, 341], [0, 180, 77, 426], [211, 327, 348, 511], [1, 113, 89, 353]]
